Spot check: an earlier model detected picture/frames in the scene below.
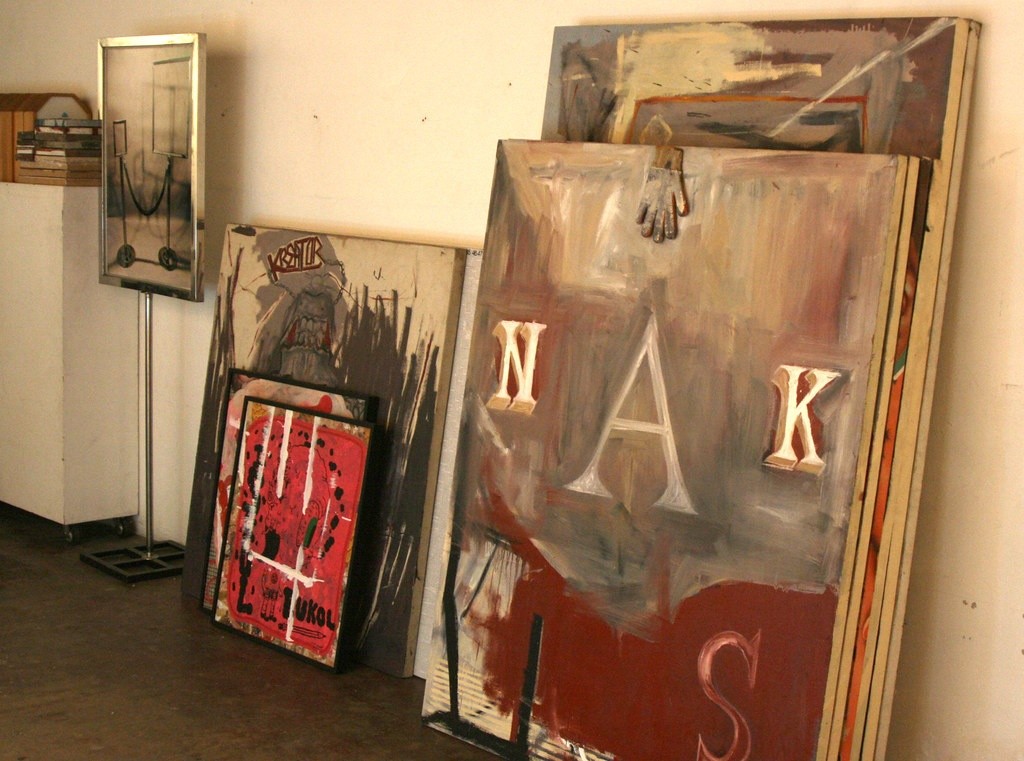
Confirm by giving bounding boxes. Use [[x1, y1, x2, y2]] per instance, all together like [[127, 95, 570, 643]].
[[93, 26, 214, 306], [199, 367, 384, 676]]
